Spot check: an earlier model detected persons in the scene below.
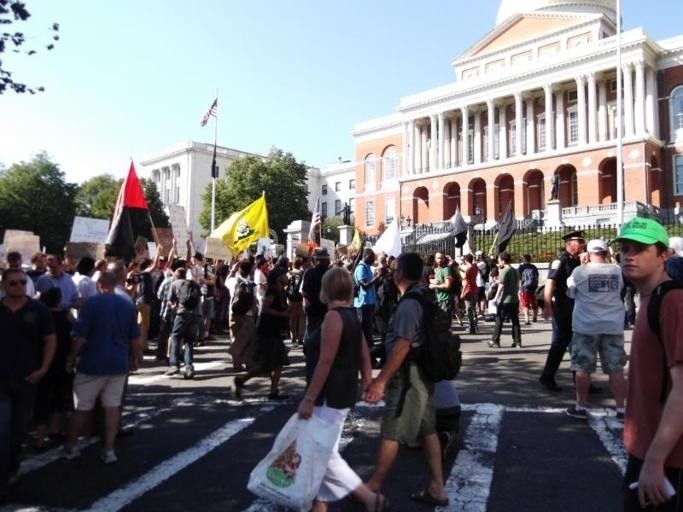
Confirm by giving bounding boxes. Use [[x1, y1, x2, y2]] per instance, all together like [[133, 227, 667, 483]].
[[298, 268, 387, 511], [1, 248, 144, 478], [539, 217, 681, 511], [459, 250, 539, 350], [124, 239, 397, 401], [365, 251, 462, 506]]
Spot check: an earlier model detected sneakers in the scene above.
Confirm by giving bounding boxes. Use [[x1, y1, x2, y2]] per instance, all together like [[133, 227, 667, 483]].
[[62, 437, 82, 461], [98, 448, 119, 465], [165, 340, 303, 402], [615, 411, 625, 422], [573, 383, 603, 394], [565, 403, 589, 420], [538, 373, 564, 393], [457, 310, 539, 350]]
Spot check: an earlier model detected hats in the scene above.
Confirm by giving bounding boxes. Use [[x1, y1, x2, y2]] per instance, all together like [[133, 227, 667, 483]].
[[607, 217, 669, 248], [669, 236, 683, 258], [586, 239, 608, 253], [561, 230, 585, 241]]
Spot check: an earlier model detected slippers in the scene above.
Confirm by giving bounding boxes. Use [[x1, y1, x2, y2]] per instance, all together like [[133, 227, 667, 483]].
[[406, 488, 450, 507]]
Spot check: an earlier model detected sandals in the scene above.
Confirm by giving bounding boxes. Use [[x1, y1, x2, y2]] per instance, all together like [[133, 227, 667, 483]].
[[364, 490, 392, 512]]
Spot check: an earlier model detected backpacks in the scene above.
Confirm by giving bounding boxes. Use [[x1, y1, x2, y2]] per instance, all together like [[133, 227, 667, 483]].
[[392, 286, 463, 384]]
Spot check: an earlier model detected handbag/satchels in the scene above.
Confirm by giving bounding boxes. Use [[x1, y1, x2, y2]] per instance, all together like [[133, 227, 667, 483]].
[[245, 411, 340, 512]]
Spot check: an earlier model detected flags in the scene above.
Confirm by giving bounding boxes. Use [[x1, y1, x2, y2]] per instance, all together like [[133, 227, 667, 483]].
[[201, 95, 217, 127], [307, 197, 322, 244], [103, 162, 148, 257], [450, 205, 468, 248], [498, 198, 517, 253], [204, 195, 270, 264]]
